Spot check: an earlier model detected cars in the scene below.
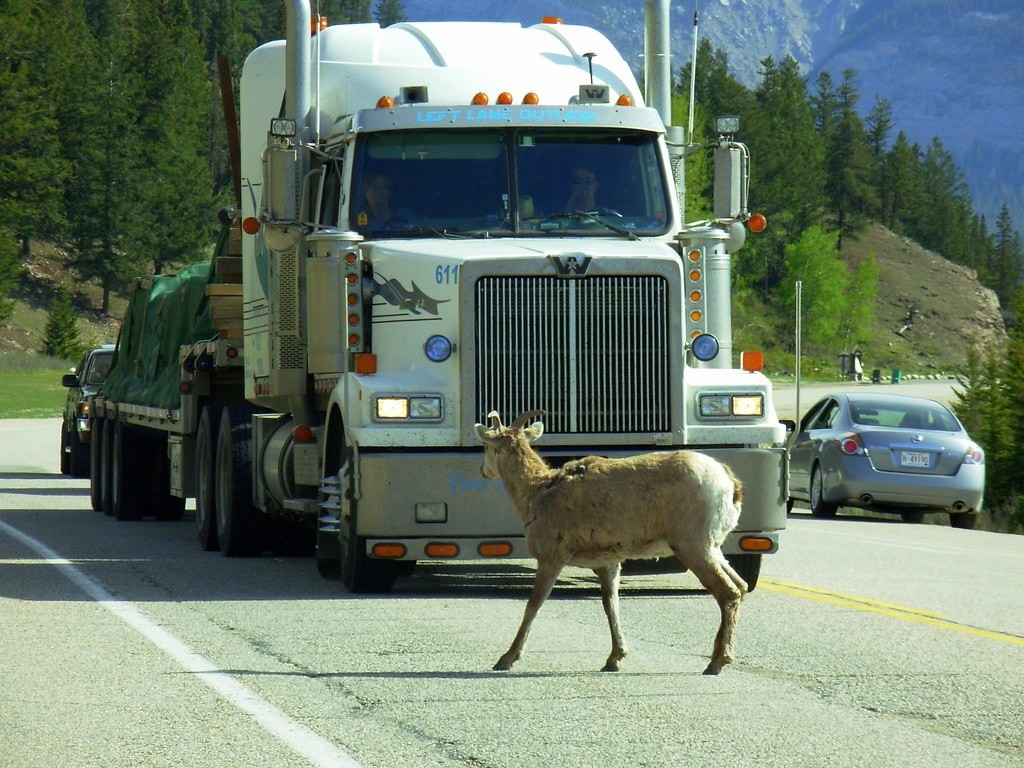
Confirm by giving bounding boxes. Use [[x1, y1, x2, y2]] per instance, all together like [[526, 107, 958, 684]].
[[782, 392, 986, 529], [59, 343, 121, 480]]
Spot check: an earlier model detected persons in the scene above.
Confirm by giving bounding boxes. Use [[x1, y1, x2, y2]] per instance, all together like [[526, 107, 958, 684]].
[[88, 364, 109, 384], [350, 171, 419, 233], [560, 165, 619, 217]]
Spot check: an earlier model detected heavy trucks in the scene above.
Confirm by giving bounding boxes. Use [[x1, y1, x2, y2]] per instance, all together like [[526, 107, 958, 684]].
[[88, 0, 803, 600]]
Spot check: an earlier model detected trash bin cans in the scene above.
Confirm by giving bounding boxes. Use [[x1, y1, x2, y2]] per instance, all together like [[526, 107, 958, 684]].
[[891, 369, 900, 384], [873, 369, 880, 384]]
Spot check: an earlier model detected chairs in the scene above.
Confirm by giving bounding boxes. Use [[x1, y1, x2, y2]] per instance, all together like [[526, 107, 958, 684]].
[[899, 409, 929, 428]]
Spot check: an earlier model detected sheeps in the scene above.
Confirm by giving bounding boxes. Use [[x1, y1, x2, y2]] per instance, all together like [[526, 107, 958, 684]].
[[472, 409, 751, 674]]
